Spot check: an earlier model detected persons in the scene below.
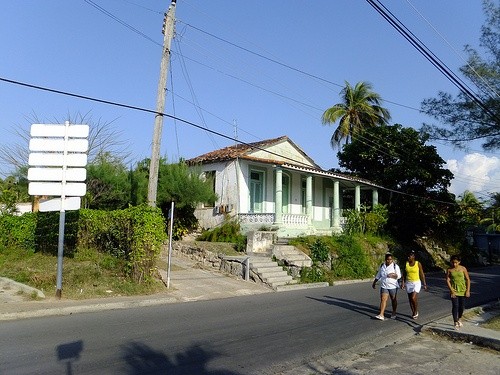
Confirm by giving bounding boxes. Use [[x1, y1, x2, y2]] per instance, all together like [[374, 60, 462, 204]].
[[371, 253, 402, 321], [446, 254, 471, 330], [400, 253, 427, 320]]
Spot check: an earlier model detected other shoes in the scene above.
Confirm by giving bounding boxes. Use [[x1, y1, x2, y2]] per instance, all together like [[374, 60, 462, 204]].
[[454, 322, 458, 330], [375, 315, 384, 321], [458, 321, 463, 327], [390, 314, 397, 319], [414, 317, 417, 319], [413, 311, 419, 317]]
